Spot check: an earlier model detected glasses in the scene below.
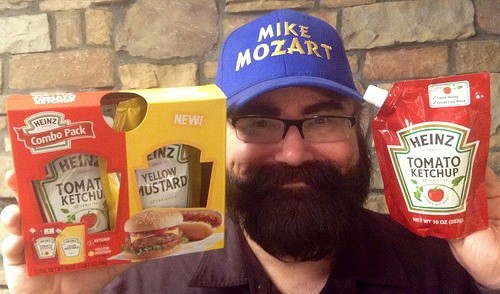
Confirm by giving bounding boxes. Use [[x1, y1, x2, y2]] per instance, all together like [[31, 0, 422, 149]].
[[227, 109, 363, 142]]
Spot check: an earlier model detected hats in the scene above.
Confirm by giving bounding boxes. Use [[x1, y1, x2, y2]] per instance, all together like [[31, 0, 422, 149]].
[[217, 9, 368, 115]]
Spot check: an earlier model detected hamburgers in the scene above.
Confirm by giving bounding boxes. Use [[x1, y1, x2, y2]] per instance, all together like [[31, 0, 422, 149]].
[[121, 207, 185, 261]]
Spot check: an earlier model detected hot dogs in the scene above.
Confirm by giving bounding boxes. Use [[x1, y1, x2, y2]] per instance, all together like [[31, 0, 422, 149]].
[[179, 208, 223, 241]]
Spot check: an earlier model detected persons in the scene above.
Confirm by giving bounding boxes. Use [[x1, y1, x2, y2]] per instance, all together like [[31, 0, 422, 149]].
[[0, 9, 500, 293]]
